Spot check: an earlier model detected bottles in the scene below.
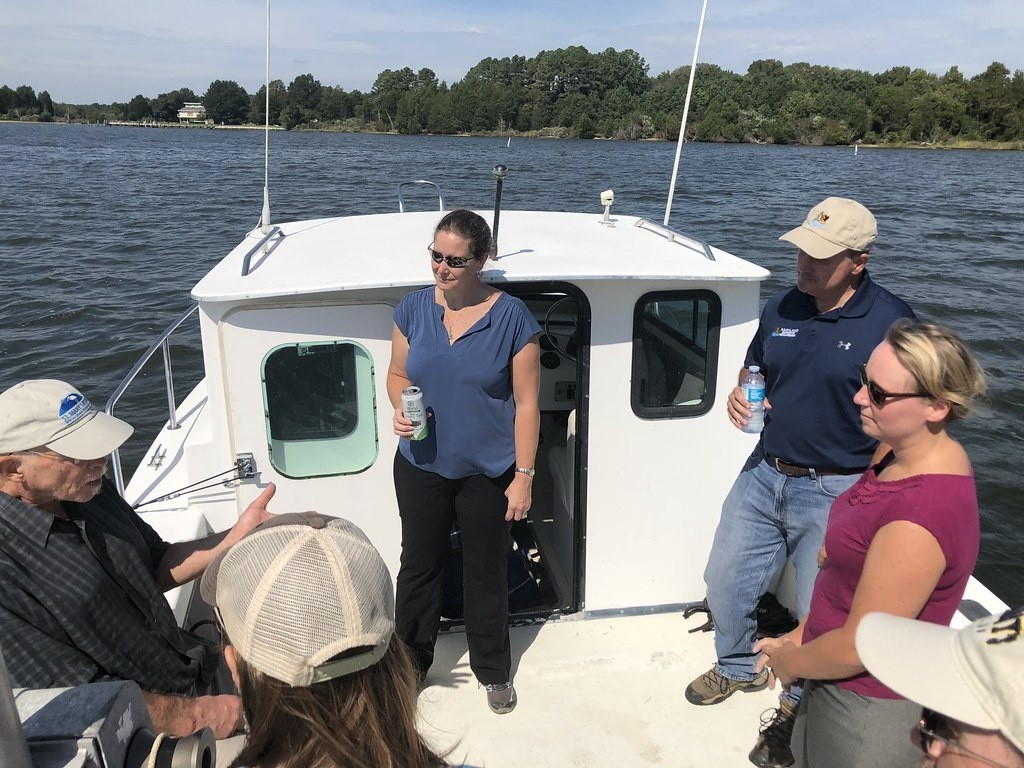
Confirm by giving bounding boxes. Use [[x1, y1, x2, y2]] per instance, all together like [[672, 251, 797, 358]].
[[740, 365, 765, 434]]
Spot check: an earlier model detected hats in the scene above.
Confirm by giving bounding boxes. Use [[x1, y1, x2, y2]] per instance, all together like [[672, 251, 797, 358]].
[[855, 608, 1024, 753], [200, 511, 395, 687], [0, 379, 134, 460], [778, 196, 877, 260]]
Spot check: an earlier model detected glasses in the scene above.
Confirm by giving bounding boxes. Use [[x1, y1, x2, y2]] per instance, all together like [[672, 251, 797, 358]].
[[428, 241, 479, 268], [8, 450, 80, 465], [919, 709, 1007, 768], [860, 362, 934, 407]]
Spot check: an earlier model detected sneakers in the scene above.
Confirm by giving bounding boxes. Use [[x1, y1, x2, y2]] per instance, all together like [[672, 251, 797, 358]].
[[748, 697, 801, 768], [477, 681, 517, 714], [685, 665, 769, 706]]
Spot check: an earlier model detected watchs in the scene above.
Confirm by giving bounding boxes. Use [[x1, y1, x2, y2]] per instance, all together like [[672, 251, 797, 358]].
[[515, 468, 535, 477]]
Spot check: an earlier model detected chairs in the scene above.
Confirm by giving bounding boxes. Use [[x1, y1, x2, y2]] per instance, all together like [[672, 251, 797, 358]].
[[548, 409, 576, 518]]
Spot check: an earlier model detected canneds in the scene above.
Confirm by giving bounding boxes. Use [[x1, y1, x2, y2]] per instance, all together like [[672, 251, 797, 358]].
[[401, 386, 429, 440]]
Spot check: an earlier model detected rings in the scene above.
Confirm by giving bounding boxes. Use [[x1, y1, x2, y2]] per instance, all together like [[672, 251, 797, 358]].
[[522, 514, 527, 517]]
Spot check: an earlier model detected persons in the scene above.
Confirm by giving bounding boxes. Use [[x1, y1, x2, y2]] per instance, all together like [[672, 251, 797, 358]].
[[386, 209, 543, 714], [0, 379, 280, 768], [856, 607, 1024, 768], [754, 317, 987, 768], [684, 197, 921, 706], [199, 510, 479, 768]]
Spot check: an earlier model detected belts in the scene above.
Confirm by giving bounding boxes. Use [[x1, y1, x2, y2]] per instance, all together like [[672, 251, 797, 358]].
[[765, 453, 865, 477]]
